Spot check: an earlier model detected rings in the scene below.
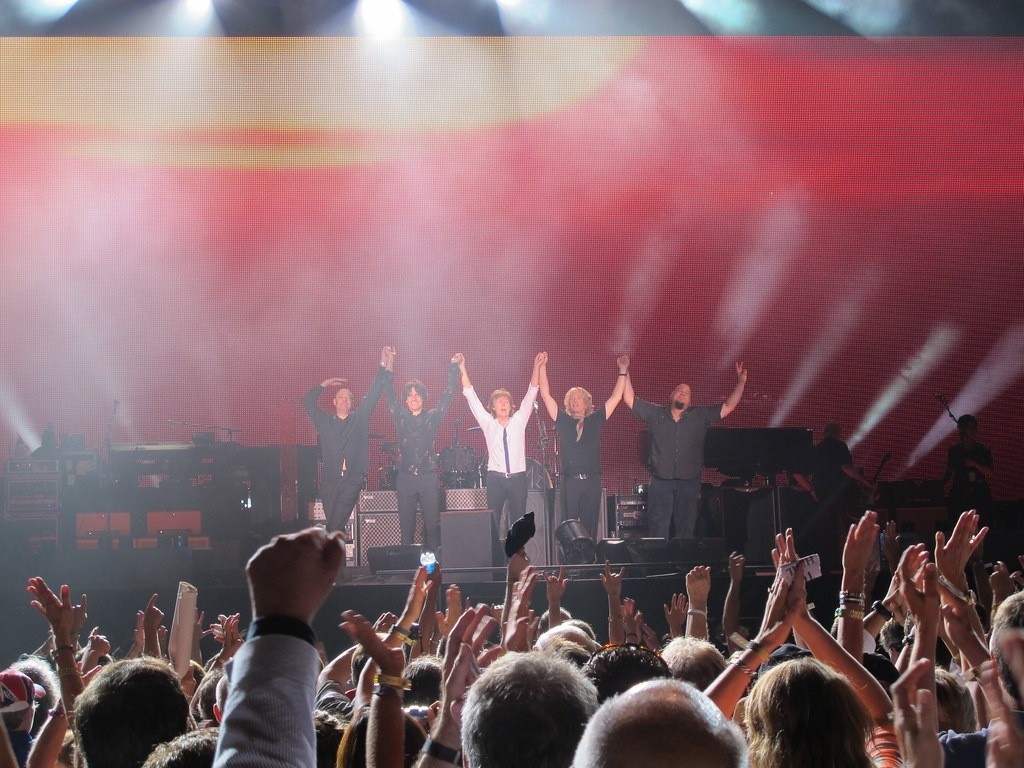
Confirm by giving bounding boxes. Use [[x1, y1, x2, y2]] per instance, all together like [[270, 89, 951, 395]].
[[768, 588, 773, 592]]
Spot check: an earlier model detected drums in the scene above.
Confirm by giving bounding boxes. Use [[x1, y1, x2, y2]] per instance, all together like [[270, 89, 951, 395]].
[[525, 457, 554, 489]]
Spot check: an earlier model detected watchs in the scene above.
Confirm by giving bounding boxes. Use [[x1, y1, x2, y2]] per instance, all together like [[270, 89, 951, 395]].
[[50, 645, 75, 660]]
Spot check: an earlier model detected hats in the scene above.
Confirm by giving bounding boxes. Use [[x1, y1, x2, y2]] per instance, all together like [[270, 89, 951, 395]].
[[757, 645, 814, 679], [863, 651, 899, 684], [0, 669, 46, 713]]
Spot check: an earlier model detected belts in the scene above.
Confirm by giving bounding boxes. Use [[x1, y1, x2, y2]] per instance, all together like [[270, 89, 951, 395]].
[[340, 470, 347, 477], [488, 470, 526, 479], [566, 472, 594, 479]]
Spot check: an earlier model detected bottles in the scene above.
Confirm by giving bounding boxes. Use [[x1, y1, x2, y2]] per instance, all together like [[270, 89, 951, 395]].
[[420, 547, 437, 575]]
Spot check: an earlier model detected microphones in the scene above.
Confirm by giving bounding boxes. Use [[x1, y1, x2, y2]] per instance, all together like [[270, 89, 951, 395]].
[[111, 398, 120, 416]]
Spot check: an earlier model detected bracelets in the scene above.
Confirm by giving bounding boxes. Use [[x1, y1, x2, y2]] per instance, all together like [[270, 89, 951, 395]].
[[373, 674, 412, 691], [688, 609, 706, 616], [214, 657, 225, 664], [381, 360, 386, 367], [953, 653, 960, 660], [371, 683, 404, 697], [421, 738, 463, 767], [618, 374, 627, 376], [902, 636, 915, 646], [608, 615, 625, 623], [729, 658, 754, 677], [48, 709, 65, 719], [391, 624, 412, 636], [746, 640, 769, 662], [390, 630, 414, 646], [835, 608, 864, 619], [838, 591, 865, 607], [626, 633, 637, 637], [56, 665, 82, 676], [871, 601, 892, 621], [809, 488, 814, 493]]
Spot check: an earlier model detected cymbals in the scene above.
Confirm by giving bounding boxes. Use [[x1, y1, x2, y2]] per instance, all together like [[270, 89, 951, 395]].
[[536, 428, 557, 432], [467, 426, 481, 431]]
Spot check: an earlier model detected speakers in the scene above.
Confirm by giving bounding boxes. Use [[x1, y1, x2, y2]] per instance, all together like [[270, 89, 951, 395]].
[[778, 486, 826, 556], [549, 488, 608, 566], [666, 537, 724, 560], [366, 544, 435, 574], [721, 484, 777, 562], [498, 490, 553, 567], [440, 510, 505, 582]]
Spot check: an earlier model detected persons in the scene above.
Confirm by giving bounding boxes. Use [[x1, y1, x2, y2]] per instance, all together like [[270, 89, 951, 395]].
[[301, 346, 747, 546], [947, 414, 994, 506], [792, 423, 877, 568], [0, 508, 1024, 768]]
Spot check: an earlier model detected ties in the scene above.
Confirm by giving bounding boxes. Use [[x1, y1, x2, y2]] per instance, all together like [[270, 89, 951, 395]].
[[502, 427, 510, 473]]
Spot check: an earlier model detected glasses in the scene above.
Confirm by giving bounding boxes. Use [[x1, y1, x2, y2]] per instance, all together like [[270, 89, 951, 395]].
[[33, 701, 41, 709], [589, 641, 672, 679]]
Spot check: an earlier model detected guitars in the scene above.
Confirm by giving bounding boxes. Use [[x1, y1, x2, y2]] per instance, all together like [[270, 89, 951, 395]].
[[857, 453, 893, 506]]
